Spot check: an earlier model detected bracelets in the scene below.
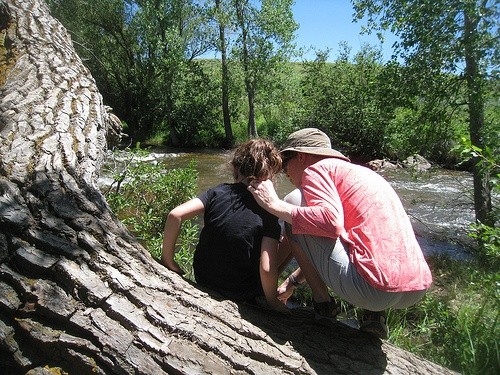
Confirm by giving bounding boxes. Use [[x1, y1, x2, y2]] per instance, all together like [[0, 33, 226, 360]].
[[288, 273, 304, 289]]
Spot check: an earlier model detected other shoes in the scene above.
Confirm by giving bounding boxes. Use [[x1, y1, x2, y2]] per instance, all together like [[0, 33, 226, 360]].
[[314, 296, 340, 320], [359, 310, 389, 340]]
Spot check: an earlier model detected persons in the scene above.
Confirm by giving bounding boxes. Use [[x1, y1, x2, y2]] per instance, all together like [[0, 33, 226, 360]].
[[246, 126, 435, 342], [160, 136, 299, 318]]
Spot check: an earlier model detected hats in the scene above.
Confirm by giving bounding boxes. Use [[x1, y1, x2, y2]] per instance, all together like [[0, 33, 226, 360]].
[[279, 128, 351, 162]]
